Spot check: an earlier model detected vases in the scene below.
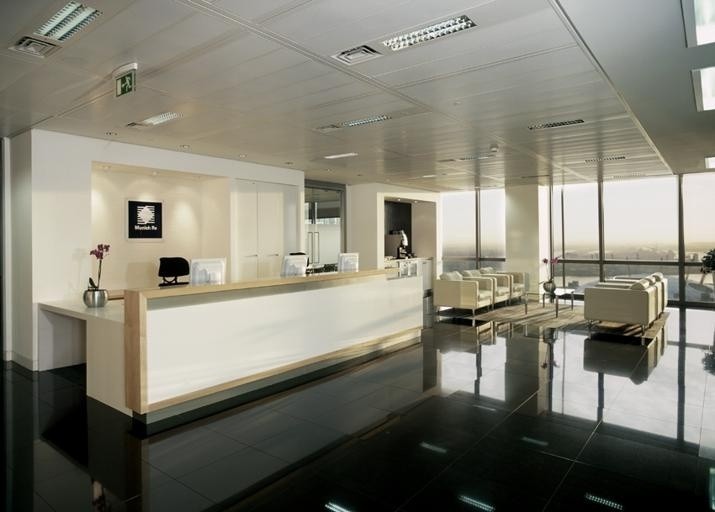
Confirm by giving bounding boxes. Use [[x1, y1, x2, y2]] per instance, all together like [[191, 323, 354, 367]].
[[544, 282, 556, 292], [84, 290, 107, 308]]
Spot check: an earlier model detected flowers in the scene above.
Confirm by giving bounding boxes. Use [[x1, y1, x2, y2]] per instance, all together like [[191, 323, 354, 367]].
[[543, 256, 558, 283], [88, 244, 110, 290]]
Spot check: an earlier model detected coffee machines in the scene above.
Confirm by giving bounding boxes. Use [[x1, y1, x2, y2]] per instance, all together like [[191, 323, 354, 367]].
[[384, 228, 413, 260]]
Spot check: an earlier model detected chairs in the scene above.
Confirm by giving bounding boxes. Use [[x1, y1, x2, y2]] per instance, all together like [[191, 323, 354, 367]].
[[463, 274, 510, 311], [482, 271, 525, 305], [606, 277, 668, 313], [599, 280, 662, 319], [584, 284, 657, 336], [159, 259, 190, 287], [433, 277, 493, 317]]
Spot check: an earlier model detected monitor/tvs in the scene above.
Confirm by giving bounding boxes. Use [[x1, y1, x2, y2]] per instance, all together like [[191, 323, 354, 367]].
[[338, 253, 359, 272], [190, 258, 226, 285], [279, 256, 308, 275]]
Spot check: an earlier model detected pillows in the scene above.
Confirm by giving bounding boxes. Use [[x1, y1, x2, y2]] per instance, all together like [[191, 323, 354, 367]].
[[481, 266, 494, 274], [645, 276, 656, 285], [630, 278, 649, 290], [463, 269, 481, 278], [652, 272, 663, 281], [439, 271, 463, 280]]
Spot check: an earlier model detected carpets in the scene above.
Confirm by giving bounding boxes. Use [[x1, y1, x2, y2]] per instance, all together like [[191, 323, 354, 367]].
[[439, 300, 670, 340]]
